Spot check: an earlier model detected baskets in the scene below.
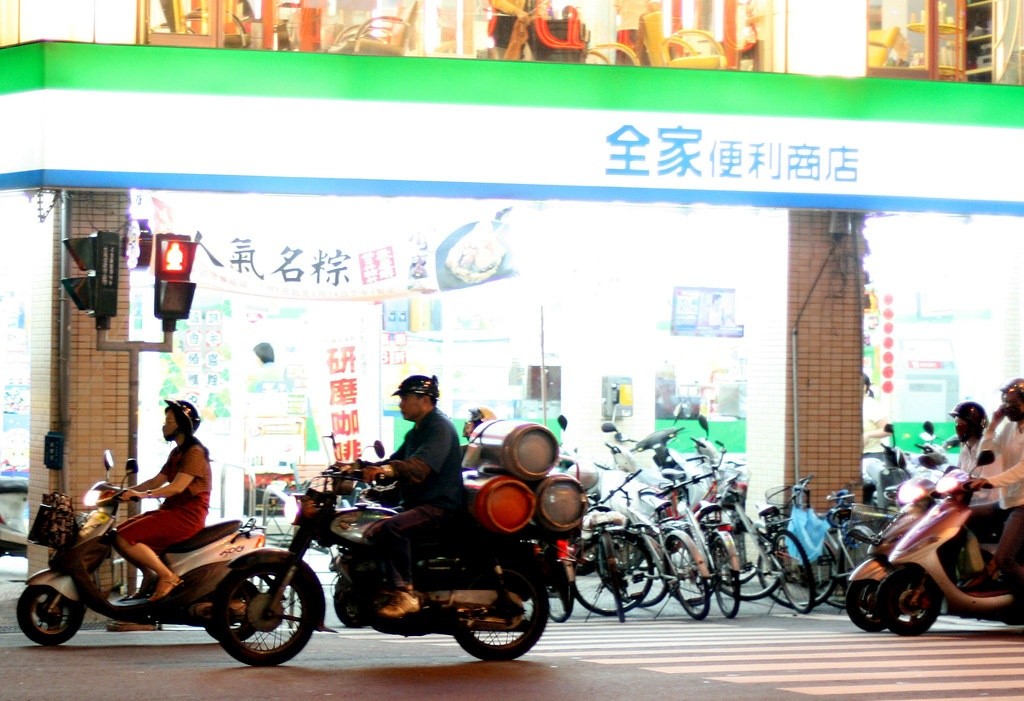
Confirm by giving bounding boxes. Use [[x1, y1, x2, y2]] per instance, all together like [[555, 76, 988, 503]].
[[26, 503, 90, 548], [846, 503, 899, 547], [765, 485, 792, 520]]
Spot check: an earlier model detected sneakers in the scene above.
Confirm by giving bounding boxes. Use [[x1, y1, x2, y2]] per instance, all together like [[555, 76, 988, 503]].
[[378, 590, 420, 618]]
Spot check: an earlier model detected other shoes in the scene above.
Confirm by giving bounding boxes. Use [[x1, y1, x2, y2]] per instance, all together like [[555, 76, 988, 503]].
[[960, 568, 996, 592]]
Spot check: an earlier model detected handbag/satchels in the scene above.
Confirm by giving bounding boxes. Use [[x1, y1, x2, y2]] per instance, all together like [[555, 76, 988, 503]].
[[36, 490, 77, 548]]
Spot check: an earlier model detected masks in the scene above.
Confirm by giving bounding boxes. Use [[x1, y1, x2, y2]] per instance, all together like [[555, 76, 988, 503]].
[[1002, 405, 1024, 421], [954, 423, 977, 442]]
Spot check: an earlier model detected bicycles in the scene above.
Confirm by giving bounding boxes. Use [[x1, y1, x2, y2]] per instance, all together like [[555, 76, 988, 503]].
[[544, 439, 878, 624]]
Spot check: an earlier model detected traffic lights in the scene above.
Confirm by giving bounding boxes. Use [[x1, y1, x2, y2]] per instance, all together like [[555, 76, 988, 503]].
[[59, 230, 119, 317], [154, 233, 198, 320]]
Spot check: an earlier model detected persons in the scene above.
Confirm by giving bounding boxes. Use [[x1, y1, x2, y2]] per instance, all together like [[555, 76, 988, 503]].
[[958, 378, 1024, 591], [112, 400, 213, 602], [488, 0, 555, 61], [613, 0, 663, 66], [462, 407, 497, 442], [708, 293, 724, 328], [863, 372, 891, 504], [335, 375, 466, 618], [948, 402, 1001, 504]]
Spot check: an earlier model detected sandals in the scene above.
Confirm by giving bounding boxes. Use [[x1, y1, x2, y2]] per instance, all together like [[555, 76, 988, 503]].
[[119, 575, 158, 600], [147, 573, 185, 602]]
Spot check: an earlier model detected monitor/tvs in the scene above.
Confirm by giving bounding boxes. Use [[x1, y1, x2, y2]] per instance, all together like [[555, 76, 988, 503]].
[[671, 286, 744, 338]]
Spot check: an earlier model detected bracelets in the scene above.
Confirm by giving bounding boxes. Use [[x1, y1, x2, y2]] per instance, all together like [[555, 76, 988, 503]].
[[147, 490, 152, 498]]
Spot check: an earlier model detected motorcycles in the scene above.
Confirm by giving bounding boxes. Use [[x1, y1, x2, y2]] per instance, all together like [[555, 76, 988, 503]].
[[209, 433, 552, 668]]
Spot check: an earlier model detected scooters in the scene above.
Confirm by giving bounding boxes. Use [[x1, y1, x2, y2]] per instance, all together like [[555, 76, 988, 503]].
[[845, 421, 1024, 637], [0, 476, 27, 558], [16, 448, 268, 646], [554, 401, 749, 521]]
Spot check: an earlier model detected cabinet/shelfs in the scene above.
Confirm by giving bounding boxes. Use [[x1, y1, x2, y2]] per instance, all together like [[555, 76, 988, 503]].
[[907, 22, 963, 83], [963, 0, 996, 83]]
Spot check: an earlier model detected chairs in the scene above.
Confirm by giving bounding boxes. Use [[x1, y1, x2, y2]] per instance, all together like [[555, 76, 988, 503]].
[[325, 23, 723, 73]]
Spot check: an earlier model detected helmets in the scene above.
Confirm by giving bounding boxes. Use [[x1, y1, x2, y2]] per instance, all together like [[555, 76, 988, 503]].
[[164, 399, 201, 434], [1001, 378, 1024, 396], [947, 401, 988, 430], [392, 373, 440, 398]]
